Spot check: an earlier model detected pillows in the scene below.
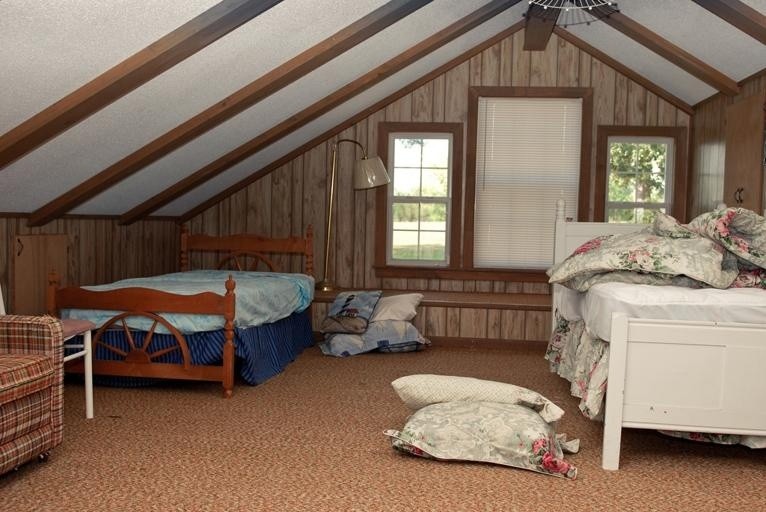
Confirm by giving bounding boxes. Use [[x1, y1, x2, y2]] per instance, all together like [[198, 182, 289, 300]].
[[316, 289, 433, 357], [384, 373, 583, 481]]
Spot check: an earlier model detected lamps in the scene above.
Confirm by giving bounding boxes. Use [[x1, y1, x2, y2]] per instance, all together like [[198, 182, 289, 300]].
[[315, 138, 390, 293]]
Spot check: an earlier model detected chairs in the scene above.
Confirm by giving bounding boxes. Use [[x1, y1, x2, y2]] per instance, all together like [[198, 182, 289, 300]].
[[1, 232, 97, 479]]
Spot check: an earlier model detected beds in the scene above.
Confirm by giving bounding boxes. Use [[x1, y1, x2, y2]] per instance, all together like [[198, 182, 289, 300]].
[[45, 219, 316, 397], [551, 189, 766, 472]]
